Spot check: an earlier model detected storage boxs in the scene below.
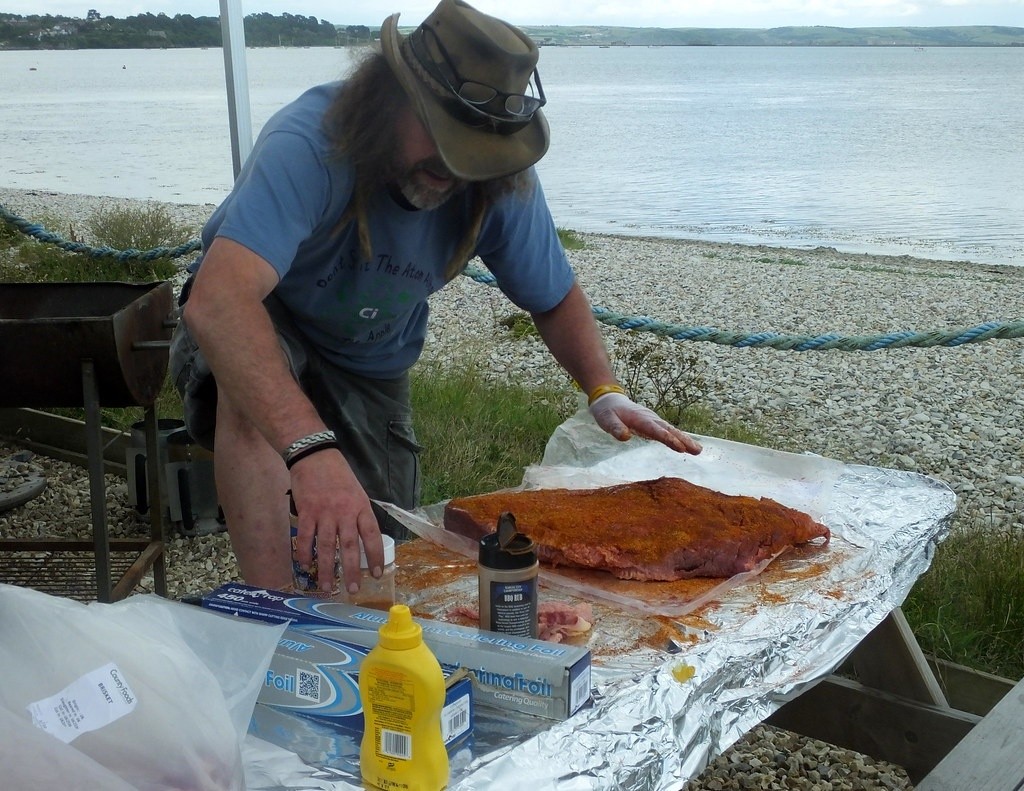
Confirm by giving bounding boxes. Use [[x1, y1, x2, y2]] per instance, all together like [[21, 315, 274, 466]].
[[175, 597, 474, 753], [206, 581, 592, 723]]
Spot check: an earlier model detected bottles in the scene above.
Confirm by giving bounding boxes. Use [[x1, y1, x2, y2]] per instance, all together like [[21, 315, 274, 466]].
[[358, 604, 449, 791], [338, 534, 395, 613], [479, 511, 538, 640]]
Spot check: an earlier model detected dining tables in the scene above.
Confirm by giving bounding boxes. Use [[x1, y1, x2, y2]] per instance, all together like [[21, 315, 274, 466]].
[[239, 431, 960, 791]]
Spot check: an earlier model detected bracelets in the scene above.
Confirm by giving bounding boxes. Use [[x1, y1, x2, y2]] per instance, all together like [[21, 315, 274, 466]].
[[282, 430, 337, 466], [588, 385, 625, 404]]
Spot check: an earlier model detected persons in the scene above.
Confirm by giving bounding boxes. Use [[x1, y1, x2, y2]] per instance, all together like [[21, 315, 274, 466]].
[[178, 0, 702, 597]]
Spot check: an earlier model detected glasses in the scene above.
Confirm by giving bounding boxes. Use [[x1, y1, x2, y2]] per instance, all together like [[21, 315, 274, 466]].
[[409, 28, 536, 136], [418, 20, 547, 117]]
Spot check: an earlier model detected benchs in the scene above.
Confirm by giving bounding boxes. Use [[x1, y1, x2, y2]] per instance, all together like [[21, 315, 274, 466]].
[[913, 675, 1023, 791]]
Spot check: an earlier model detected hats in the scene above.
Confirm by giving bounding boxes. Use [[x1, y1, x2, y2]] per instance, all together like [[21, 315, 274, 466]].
[[380, 0, 552, 184]]
